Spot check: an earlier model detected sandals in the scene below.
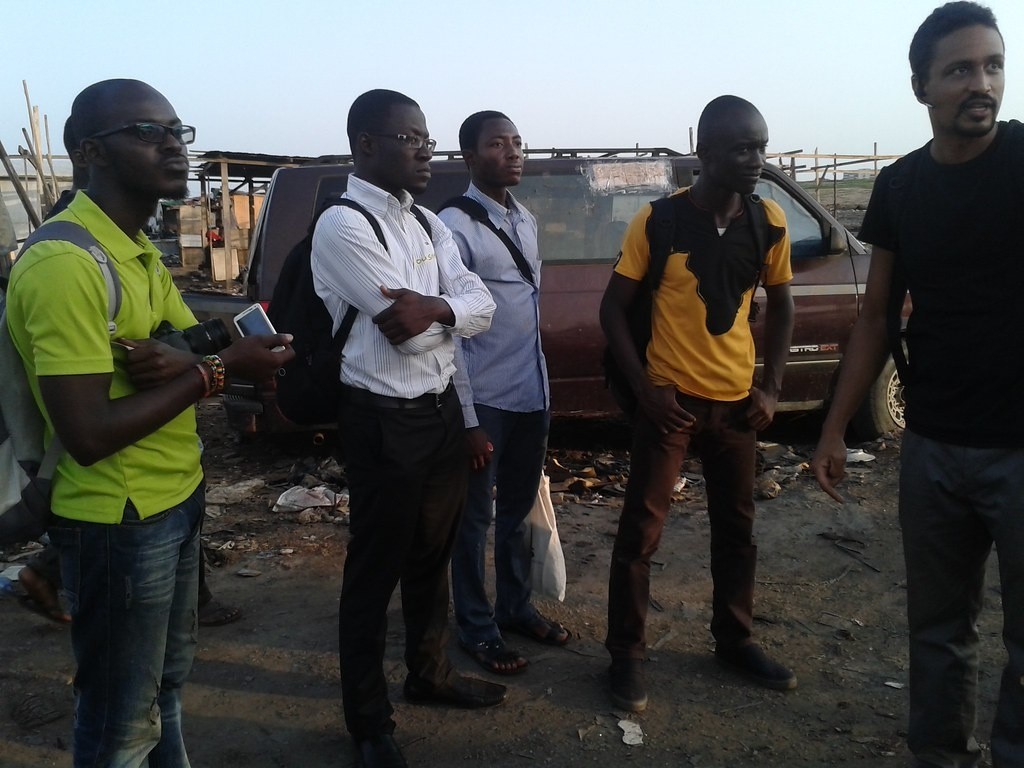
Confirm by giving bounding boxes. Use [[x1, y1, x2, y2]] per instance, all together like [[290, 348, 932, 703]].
[[198, 596, 242, 626], [16, 589, 72, 626], [456, 632, 529, 675], [494, 609, 572, 644]]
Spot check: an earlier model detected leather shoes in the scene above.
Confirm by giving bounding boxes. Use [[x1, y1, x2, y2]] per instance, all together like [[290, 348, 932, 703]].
[[608, 663, 648, 711], [358, 732, 408, 768], [714, 643, 798, 690], [404, 671, 508, 708]]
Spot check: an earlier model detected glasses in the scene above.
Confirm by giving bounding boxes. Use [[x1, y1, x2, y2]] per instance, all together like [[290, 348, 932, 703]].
[[369, 132, 437, 152], [89, 125, 196, 145]]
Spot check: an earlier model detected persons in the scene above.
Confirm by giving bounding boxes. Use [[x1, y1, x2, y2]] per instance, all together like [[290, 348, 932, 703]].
[[595, 94, 799, 704], [438, 112, 574, 675], [5, 79, 298, 767], [812, 2, 1023, 768], [311, 87, 511, 766]]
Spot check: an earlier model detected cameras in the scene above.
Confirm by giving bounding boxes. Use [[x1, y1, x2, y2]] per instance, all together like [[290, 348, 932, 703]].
[[150, 316, 232, 357]]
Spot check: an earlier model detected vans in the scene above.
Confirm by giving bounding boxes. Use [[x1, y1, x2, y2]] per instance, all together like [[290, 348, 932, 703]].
[[246, 152, 911, 471]]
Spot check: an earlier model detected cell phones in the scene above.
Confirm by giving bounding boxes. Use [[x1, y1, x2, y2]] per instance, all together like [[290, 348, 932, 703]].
[[233, 302, 286, 353]]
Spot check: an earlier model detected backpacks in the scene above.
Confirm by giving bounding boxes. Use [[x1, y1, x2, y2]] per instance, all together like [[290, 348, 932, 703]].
[[265, 198, 434, 423], [604, 192, 769, 420], [0, 220, 122, 544]]
